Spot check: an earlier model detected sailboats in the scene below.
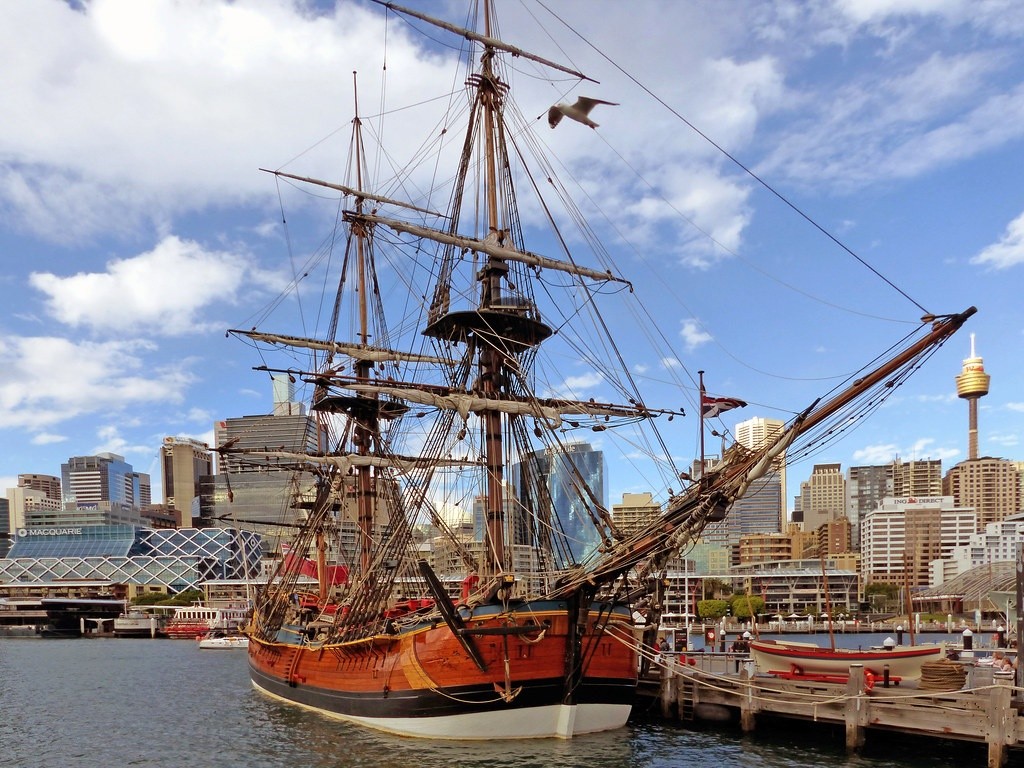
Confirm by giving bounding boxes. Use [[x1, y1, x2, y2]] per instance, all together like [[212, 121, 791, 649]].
[[748, 551, 947, 687], [225, 1, 980, 741]]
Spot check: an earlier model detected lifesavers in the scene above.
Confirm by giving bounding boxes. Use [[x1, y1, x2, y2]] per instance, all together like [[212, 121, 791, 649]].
[[862, 669, 874, 692]]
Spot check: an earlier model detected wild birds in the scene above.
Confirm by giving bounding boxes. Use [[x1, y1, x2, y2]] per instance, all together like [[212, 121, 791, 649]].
[[548, 96, 620, 129]]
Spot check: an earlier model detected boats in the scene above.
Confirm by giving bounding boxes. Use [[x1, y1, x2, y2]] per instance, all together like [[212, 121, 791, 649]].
[[166, 606, 249, 639], [199, 637, 249, 649]]
